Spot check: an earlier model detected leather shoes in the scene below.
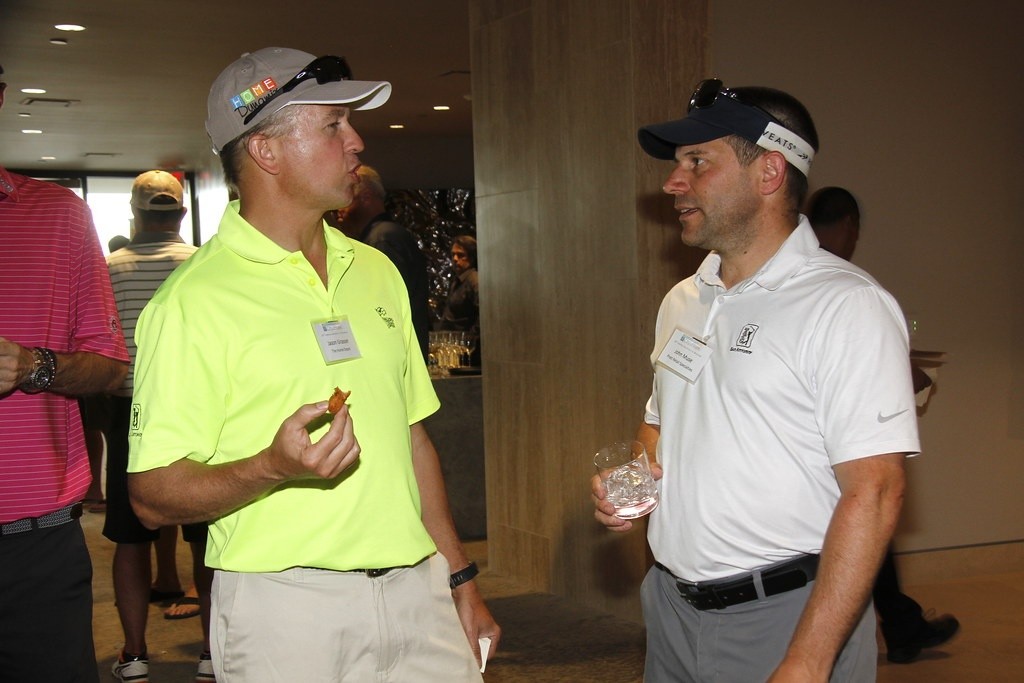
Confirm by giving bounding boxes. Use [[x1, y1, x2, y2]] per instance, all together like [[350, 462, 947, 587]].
[[886, 612, 959, 664]]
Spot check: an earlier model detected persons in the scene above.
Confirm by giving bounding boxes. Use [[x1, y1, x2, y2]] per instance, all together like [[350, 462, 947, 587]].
[[0, 168, 214, 680], [126, 46, 503, 683], [332, 165, 429, 367], [590, 79, 959, 683], [438, 236, 481, 367]]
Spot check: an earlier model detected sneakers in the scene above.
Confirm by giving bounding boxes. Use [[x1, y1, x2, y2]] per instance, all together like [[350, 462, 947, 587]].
[[194, 651, 216, 683], [111, 647, 150, 683]]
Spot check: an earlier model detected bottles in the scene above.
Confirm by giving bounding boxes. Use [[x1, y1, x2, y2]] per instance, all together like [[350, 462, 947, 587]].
[[428, 332, 470, 379]]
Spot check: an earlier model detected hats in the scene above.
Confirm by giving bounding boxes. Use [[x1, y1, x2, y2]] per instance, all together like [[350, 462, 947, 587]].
[[205, 45, 392, 155], [637, 93, 816, 177], [129, 170, 184, 211]]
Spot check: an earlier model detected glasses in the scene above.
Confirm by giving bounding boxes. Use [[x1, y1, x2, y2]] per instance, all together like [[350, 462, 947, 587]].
[[243, 55, 356, 126], [686, 79, 789, 133]]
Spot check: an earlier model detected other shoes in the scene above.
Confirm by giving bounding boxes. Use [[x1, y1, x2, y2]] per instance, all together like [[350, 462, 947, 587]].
[[84, 497, 108, 513]]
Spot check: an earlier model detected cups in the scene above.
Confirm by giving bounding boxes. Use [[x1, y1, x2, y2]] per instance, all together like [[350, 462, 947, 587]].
[[907, 319, 918, 338], [593, 441, 660, 520]]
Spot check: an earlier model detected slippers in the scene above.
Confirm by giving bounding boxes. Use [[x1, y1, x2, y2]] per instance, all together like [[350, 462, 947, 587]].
[[150, 585, 185, 600], [163, 592, 201, 620]]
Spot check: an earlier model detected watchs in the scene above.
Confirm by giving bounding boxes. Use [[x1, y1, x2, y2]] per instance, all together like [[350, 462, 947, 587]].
[[450, 560, 480, 587], [19, 346, 55, 394]]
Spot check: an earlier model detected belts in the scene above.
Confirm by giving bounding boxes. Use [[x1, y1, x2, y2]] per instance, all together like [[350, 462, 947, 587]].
[[351, 566, 392, 578], [654, 553, 823, 612], [0, 502, 86, 536]]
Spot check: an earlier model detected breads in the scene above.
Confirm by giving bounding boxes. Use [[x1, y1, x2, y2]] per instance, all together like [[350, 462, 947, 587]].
[[329, 387, 352, 414]]
